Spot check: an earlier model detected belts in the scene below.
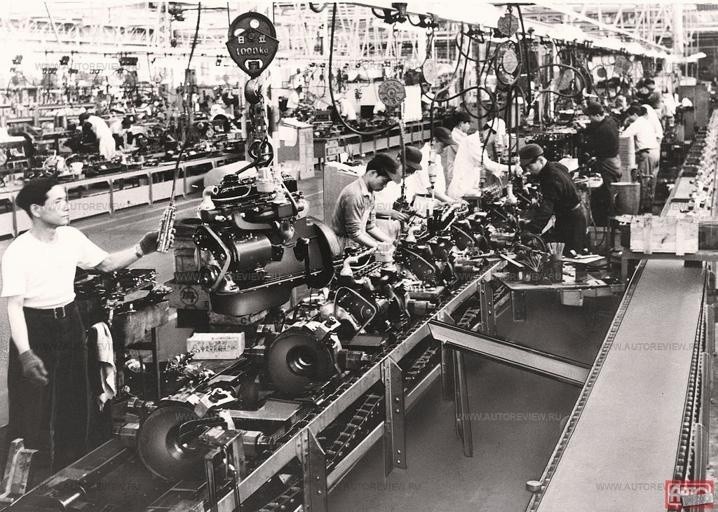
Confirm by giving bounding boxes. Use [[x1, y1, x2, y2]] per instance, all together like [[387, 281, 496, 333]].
[[637, 148, 653, 153], [558, 199, 586, 217], [23, 299, 75, 321]]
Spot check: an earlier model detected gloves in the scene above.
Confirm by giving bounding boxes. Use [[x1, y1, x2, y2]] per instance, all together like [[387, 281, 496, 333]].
[[138, 228, 176, 253], [17, 349, 47, 386]]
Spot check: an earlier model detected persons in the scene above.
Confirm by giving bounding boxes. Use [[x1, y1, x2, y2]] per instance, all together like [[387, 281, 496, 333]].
[[79, 86, 302, 159], [0, 179, 176, 490], [330, 77, 678, 256]]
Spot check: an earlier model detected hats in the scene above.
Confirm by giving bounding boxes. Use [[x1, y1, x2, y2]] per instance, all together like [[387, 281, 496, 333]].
[[401, 147, 423, 171], [457, 111, 475, 123], [433, 126, 458, 146], [14, 170, 61, 211], [369, 153, 402, 185], [78, 112, 89, 126], [519, 143, 545, 167], [582, 102, 604, 115], [625, 79, 655, 116]]
